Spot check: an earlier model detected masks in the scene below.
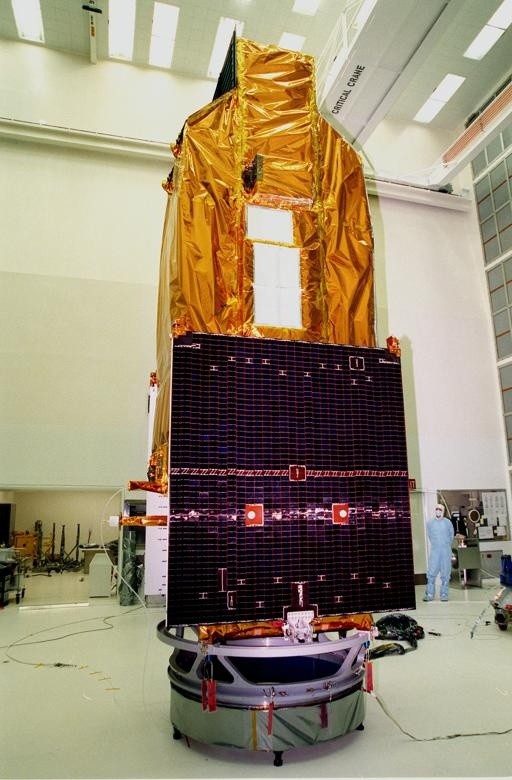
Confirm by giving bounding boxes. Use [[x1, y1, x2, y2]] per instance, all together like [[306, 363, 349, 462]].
[[435, 510, 441, 517]]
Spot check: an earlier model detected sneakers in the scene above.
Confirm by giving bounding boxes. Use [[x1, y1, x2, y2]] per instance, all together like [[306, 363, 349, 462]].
[[423, 593, 434, 601], [440, 593, 449, 601]]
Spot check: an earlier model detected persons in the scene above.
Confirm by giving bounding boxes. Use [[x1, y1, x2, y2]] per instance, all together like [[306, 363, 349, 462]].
[[422, 503, 455, 603]]
